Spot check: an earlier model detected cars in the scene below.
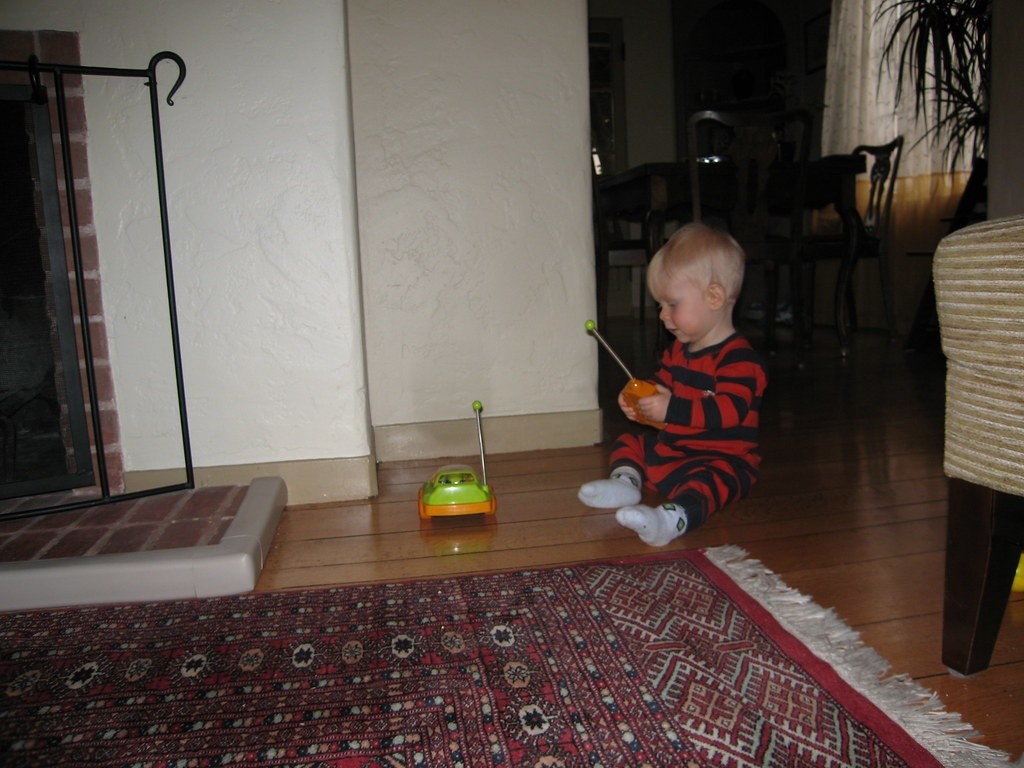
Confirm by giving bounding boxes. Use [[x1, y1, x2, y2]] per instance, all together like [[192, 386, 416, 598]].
[[417, 464, 497, 521]]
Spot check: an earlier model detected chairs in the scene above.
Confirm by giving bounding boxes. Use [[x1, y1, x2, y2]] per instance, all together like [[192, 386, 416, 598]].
[[768, 134, 903, 354], [688, 111, 813, 370], [594, 121, 667, 352], [932, 213, 1024, 677]]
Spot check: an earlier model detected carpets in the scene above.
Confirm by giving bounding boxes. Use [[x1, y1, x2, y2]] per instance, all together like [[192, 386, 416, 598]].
[[0, 545, 1013, 768]]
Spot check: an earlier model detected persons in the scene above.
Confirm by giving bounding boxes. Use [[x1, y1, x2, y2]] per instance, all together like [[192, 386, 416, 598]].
[[577, 222, 767, 547]]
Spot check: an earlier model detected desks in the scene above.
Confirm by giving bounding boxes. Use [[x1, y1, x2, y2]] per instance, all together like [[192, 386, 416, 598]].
[[601, 154, 867, 359]]
